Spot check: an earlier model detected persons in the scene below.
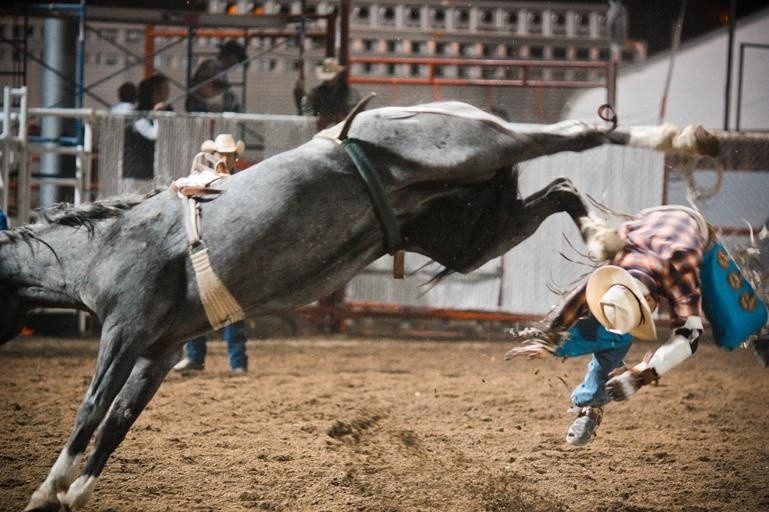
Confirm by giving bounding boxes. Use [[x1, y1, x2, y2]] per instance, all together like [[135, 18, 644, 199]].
[[170, 132, 250, 375], [303, 57, 363, 130], [107, 40, 248, 180], [505, 204, 769, 448]]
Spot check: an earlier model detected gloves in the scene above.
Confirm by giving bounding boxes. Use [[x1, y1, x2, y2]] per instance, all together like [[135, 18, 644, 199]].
[[603, 363, 659, 404]]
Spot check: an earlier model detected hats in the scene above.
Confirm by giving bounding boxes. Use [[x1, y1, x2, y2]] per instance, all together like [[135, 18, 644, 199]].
[[199, 133, 246, 155], [584, 264, 659, 344], [313, 56, 352, 83]]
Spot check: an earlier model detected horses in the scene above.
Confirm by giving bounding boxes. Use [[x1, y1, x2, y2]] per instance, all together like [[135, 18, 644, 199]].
[[0, 93, 724, 512]]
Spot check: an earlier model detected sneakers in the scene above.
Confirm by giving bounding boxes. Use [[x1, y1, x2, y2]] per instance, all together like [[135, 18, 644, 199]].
[[565, 405, 605, 448], [170, 357, 207, 373], [227, 362, 251, 377]]
